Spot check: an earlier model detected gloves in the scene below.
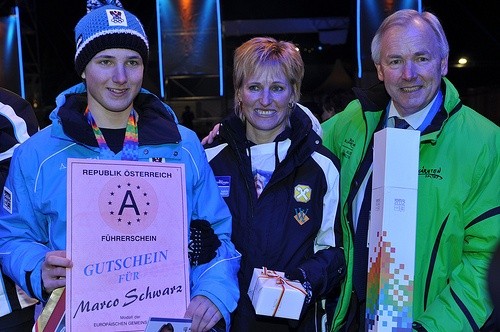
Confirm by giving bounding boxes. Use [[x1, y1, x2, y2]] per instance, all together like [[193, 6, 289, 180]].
[[188, 219, 222, 266], [285, 268, 313, 303]]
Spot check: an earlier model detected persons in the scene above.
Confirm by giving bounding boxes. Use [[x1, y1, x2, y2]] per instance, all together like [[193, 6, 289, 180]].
[[182, 105, 195, 130], [200, 9, 500, 332], [202, 38, 347, 332], [0, 87, 37, 332], [321, 94, 349, 122], [0, 0, 242, 332]]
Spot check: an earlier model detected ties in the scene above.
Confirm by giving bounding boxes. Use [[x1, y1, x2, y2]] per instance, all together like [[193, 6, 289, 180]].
[[348, 116, 410, 332]]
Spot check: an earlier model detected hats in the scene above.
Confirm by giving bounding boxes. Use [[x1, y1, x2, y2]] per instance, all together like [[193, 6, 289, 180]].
[[74, 0, 149, 78]]
